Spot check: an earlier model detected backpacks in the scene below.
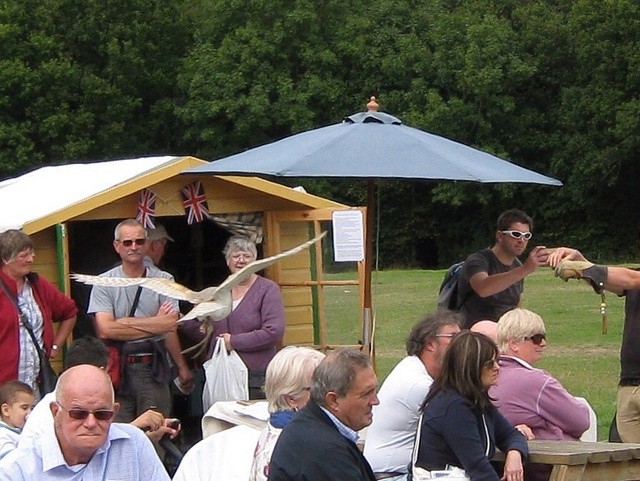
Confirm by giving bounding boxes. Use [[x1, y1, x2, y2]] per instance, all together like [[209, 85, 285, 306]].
[[437, 248, 525, 316]]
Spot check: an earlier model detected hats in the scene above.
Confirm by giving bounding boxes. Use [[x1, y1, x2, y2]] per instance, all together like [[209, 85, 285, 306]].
[[146, 224, 175, 242]]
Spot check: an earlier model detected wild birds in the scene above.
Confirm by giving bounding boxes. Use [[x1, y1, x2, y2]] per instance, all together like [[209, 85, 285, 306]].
[[66, 231, 329, 336]]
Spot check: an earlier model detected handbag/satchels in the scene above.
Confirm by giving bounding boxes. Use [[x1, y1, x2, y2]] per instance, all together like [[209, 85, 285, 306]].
[[412, 465, 471, 481], [38, 355, 58, 399], [107, 345, 127, 396]]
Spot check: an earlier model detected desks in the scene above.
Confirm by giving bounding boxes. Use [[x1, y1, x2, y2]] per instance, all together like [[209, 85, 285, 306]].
[[492, 438, 640, 481]]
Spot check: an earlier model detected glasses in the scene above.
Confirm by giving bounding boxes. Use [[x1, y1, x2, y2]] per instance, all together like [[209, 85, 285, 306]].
[[501, 229, 532, 240], [231, 253, 254, 262], [56, 401, 114, 421], [482, 354, 500, 369], [116, 237, 147, 247], [524, 334, 546, 345], [436, 333, 455, 337]]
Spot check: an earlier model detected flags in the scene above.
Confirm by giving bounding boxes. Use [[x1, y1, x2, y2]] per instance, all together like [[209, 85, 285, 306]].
[[134, 189, 156, 230], [180, 181, 210, 225]]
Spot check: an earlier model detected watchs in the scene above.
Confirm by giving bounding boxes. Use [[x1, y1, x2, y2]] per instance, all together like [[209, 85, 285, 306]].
[[51, 344, 60, 351]]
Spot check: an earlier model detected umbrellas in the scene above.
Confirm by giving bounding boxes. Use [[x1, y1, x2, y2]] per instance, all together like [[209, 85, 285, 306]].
[[177, 94, 565, 352]]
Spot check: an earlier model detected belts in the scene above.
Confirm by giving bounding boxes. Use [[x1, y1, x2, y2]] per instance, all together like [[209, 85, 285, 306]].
[[373, 472, 406, 480], [127, 355, 153, 364]]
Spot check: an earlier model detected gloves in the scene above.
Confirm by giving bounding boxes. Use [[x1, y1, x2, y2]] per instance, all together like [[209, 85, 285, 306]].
[[554, 261, 608, 294]]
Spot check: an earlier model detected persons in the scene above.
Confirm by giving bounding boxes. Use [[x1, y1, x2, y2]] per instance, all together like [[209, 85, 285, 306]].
[[142, 226, 197, 392], [0, 380, 35, 461], [457, 208, 551, 332], [250, 344, 326, 481], [0, 363, 174, 481], [362, 306, 462, 481], [85, 217, 181, 466], [184, 235, 287, 401], [0, 228, 78, 406], [545, 246, 640, 445], [406, 329, 530, 481], [487, 306, 591, 442], [18, 335, 182, 450], [265, 348, 377, 481]]
